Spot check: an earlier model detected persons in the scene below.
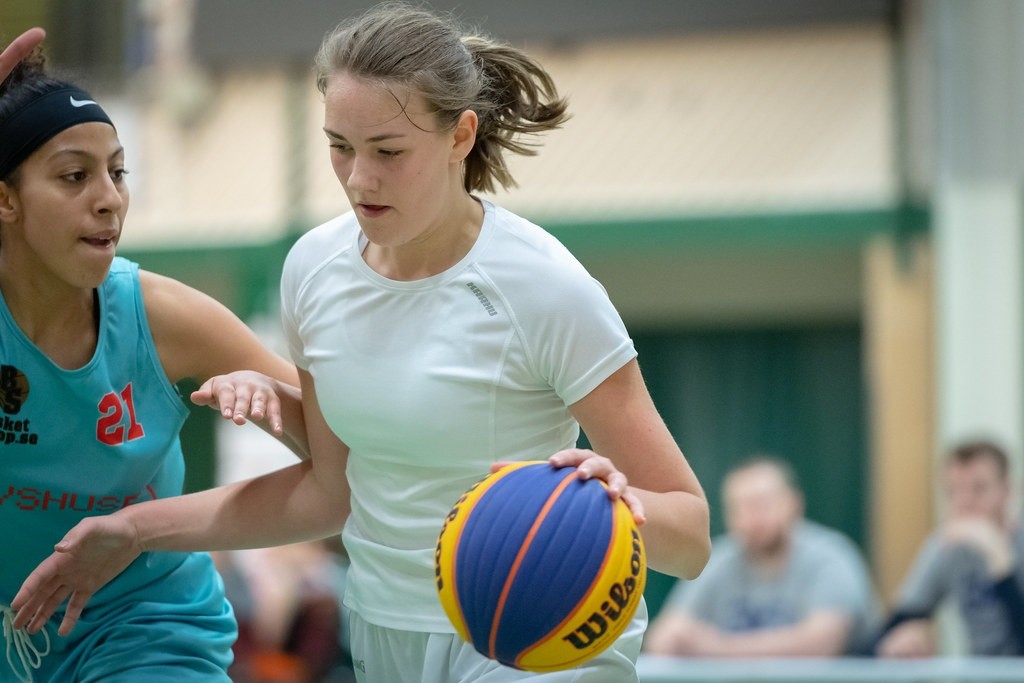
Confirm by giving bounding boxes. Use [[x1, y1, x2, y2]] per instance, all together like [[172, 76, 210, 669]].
[[9, 9, 711, 682], [0, 28, 312, 683], [213, 538, 352, 683], [864, 444, 1024, 659], [644, 457, 884, 657]]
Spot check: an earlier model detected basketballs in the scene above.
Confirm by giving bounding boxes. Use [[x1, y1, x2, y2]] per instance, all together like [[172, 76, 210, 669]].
[[434, 460, 648, 674]]
